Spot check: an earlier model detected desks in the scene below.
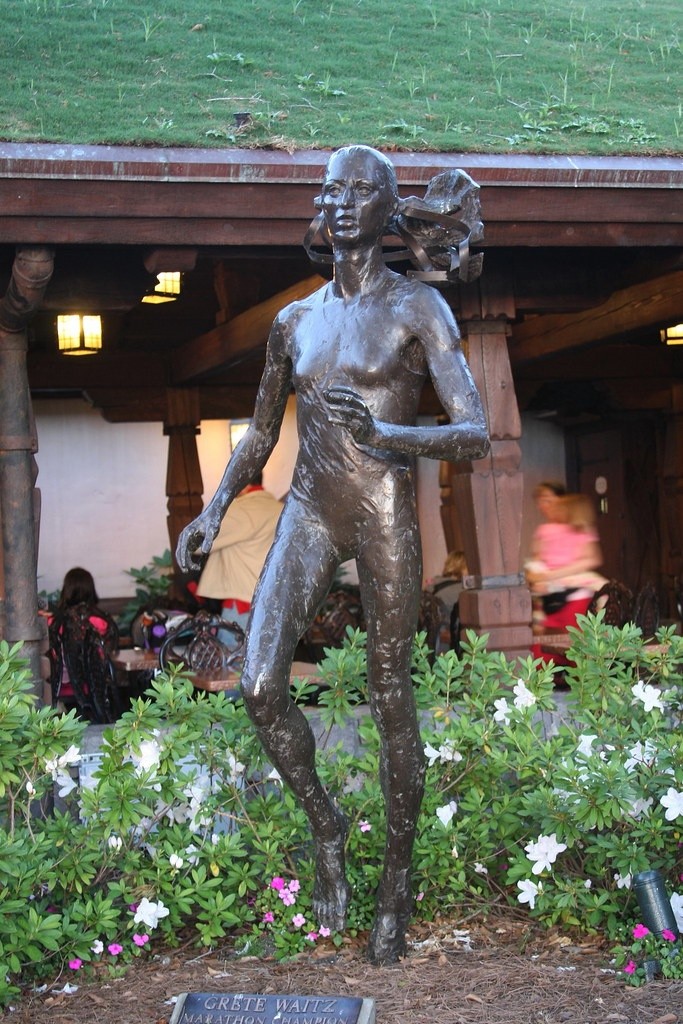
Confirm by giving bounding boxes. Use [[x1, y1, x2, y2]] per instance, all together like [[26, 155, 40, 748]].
[[533, 626, 683, 683], [189, 661, 332, 691], [110, 646, 198, 701]]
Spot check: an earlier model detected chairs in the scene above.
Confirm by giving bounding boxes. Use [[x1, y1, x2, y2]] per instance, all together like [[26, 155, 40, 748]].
[[48, 580, 483, 723], [585, 580, 660, 646]]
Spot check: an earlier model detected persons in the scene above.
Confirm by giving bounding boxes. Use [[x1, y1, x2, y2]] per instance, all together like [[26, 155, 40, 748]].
[[170, 145, 487, 961], [32, 473, 598, 710]]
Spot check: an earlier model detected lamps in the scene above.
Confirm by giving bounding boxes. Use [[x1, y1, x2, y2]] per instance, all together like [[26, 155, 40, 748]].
[[632, 870, 679, 943], [660, 322, 683, 346], [55, 314, 102, 357], [142, 271, 181, 304]]
